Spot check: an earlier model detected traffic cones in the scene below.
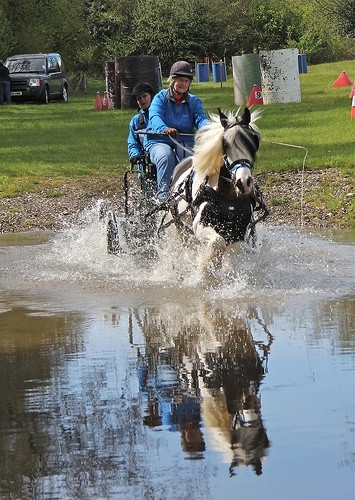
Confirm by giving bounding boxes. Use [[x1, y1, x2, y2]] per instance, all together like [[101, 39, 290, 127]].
[[93, 91, 115, 111], [350, 88, 355, 118]]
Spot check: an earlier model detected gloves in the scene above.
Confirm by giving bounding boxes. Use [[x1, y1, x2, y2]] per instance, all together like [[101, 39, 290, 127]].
[[129, 155, 142, 165], [164, 127, 178, 139]]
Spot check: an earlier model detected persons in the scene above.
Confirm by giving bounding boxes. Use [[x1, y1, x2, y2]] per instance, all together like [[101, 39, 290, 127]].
[[0, 59, 12, 104], [127, 81, 155, 163], [143, 61, 211, 201]]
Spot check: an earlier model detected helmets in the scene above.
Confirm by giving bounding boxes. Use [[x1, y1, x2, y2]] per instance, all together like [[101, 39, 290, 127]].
[[170, 61, 193, 80], [130, 82, 152, 97], [179, 429, 206, 452]]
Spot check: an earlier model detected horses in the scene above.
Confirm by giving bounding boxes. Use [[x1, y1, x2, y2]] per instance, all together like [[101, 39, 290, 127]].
[[150, 101, 263, 286], [153, 300, 272, 479]]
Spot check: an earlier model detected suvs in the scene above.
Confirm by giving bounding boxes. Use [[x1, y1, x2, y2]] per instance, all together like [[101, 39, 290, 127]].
[[4, 52, 70, 105]]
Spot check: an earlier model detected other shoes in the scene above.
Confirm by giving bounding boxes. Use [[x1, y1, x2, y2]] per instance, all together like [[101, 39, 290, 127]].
[[157, 192, 169, 202]]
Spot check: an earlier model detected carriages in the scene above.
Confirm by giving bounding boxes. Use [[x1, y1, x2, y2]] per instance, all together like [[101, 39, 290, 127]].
[[103, 103, 274, 281], [114, 296, 276, 478]]
